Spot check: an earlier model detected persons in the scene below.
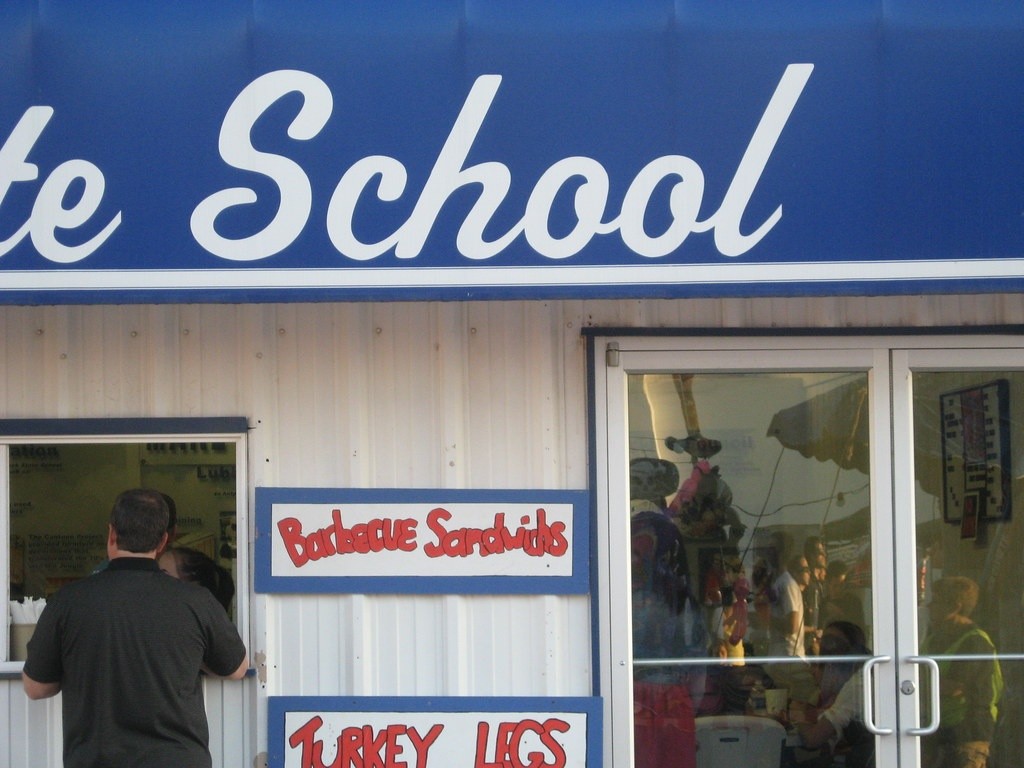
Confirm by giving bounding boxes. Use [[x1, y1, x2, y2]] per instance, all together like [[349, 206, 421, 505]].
[[632, 476, 1003, 767], [158, 546, 234, 613], [21, 488, 249, 768]]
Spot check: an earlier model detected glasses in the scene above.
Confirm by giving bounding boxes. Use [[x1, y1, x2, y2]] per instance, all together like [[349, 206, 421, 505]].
[[798, 567, 811, 574]]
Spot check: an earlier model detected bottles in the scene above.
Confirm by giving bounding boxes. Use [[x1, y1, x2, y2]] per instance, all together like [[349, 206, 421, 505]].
[[719, 585, 746, 667]]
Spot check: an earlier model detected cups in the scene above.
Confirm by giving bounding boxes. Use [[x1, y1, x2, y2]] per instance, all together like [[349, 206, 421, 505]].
[[765, 689, 787, 721], [11, 623, 37, 660]]
